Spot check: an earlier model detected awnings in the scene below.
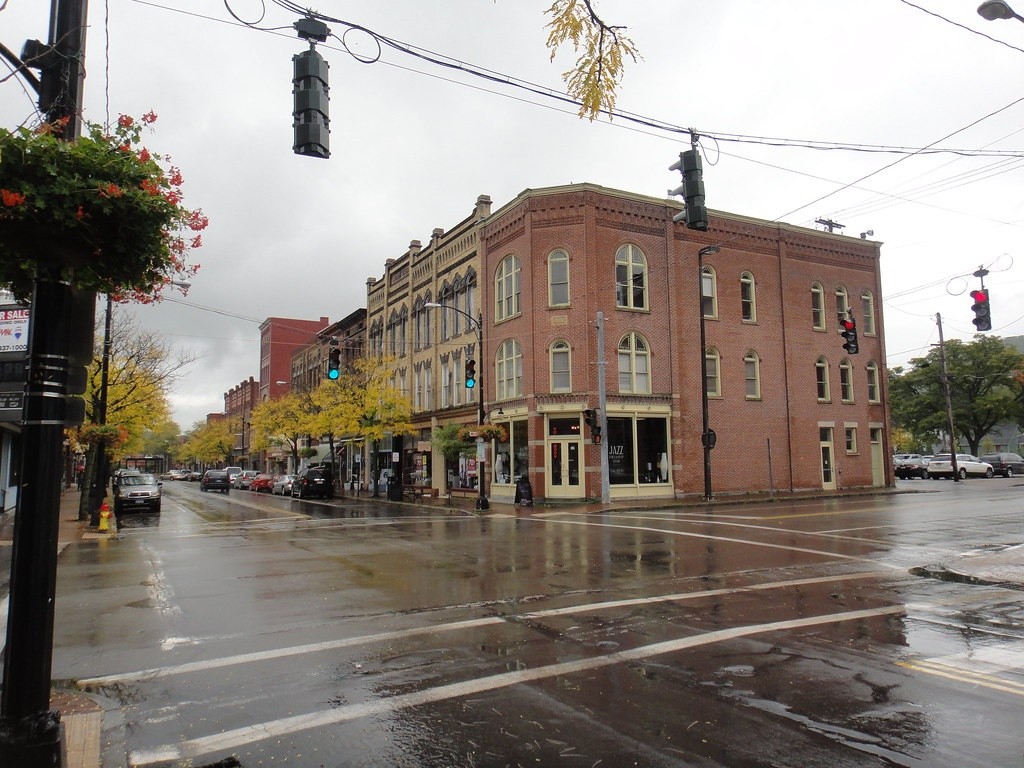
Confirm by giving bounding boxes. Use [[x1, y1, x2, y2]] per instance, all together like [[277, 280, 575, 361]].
[[303, 443, 340, 463]]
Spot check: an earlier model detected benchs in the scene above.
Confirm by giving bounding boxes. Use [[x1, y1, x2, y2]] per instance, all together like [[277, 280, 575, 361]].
[[399, 485, 424, 503]]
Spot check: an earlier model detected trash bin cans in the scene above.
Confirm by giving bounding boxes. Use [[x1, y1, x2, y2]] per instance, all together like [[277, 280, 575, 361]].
[[387, 476, 400, 500]]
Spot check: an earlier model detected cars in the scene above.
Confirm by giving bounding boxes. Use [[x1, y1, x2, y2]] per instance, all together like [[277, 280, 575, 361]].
[[171, 469, 202, 482], [114, 469, 163, 514], [159, 472, 184, 481], [249, 474, 296, 496], [897, 458, 931, 479]]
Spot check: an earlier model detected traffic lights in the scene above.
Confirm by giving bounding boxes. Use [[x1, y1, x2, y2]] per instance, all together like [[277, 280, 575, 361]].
[[840, 318, 858, 354], [592, 427, 601, 444], [970, 289, 992, 331], [584, 409, 596, 426], [464, 359, 476, 388], [328, 341, 341, 379]]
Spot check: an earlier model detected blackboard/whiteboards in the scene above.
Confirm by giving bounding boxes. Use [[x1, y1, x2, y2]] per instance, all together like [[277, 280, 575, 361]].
[[515, 482, 532, 503]]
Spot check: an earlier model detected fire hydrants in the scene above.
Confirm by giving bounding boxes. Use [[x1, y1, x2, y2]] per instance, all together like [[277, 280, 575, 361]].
[[97, 503, 113, 532]]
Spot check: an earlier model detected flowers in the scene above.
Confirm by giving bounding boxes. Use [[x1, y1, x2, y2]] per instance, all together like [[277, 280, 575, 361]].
[[455, 425, 508, 444], [0, 109, 210, 307], [71, 421, 130, 450]]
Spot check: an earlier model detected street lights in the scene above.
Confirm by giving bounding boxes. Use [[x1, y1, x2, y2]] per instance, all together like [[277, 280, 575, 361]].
[[699, 246, 722, 499], [423, 302, 504, 509]]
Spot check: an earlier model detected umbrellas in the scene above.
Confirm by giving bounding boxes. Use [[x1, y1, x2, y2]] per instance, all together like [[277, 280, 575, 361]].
[[73, 466, 85, 471]]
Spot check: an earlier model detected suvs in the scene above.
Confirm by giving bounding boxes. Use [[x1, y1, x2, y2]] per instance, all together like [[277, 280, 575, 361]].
[[222, 467, 261, 490], [980, 452, 1024, 478], [893, 453, 924, 472], [926, 454, 995, 480], [291, 468, 335, 499], [200, 469, 230, 493]]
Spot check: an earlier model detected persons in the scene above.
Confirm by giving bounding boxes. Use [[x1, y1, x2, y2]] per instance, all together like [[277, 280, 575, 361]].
[[77, 470, 84, 490]]
